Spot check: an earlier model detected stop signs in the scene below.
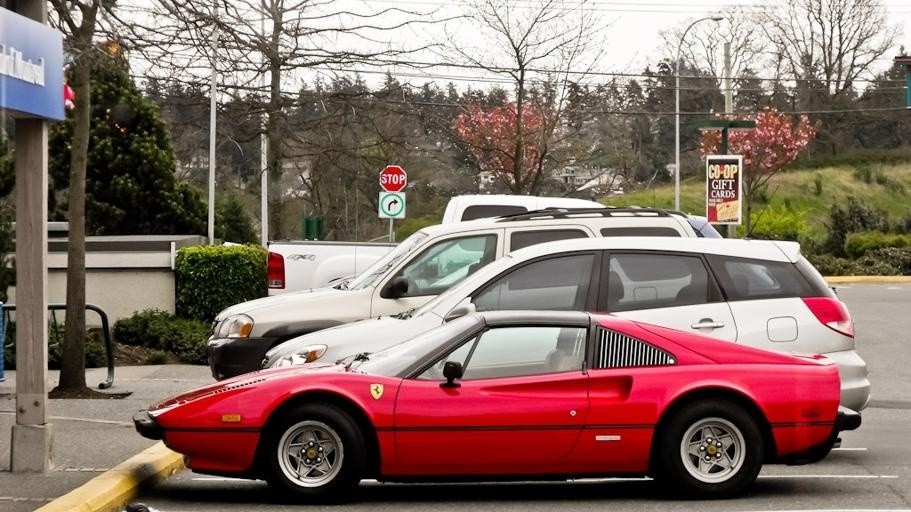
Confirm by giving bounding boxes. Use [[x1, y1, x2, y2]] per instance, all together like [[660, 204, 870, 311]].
[[379, 164, 407, 192]]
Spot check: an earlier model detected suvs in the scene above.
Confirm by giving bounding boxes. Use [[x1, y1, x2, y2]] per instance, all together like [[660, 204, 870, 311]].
[[258, 237, 869, 422], [202, 204, 727, 383]]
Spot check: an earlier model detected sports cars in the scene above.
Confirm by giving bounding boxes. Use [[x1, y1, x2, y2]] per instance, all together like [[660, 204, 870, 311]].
[[127, 310, 861, 495]]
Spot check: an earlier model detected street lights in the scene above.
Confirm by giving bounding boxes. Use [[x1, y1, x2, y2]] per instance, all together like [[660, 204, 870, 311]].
[[694, 117, 756, 240], [669, 14, 729, 211]]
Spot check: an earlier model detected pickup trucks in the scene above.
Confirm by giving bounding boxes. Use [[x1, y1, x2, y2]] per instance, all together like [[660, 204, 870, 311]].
[[259, 190, 616, 302]]
